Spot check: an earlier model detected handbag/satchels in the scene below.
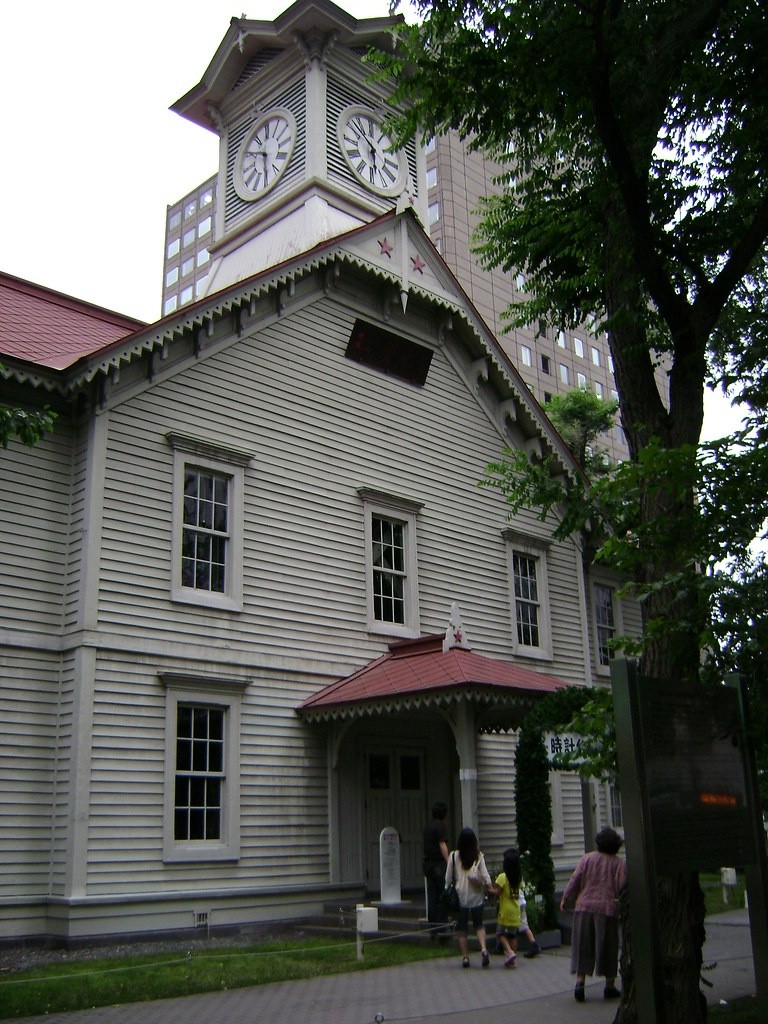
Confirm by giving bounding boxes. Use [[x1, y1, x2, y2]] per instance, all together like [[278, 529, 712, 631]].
[[468, 851, 486, 887], [444, 884, 460, 912]]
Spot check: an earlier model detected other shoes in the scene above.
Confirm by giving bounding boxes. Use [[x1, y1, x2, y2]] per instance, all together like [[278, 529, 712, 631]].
[[604, 986, 620, 1000], [463, 957, 470, 968], [502, 954, 518, 968], [482, 950, 489, 967], [574, 983, 585, 1002], [524, 948, 542, 958]]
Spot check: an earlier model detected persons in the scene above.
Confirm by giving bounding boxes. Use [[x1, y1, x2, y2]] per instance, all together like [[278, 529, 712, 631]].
[[559, 829, 627, 1004], [424, 802, 541, 969]]
